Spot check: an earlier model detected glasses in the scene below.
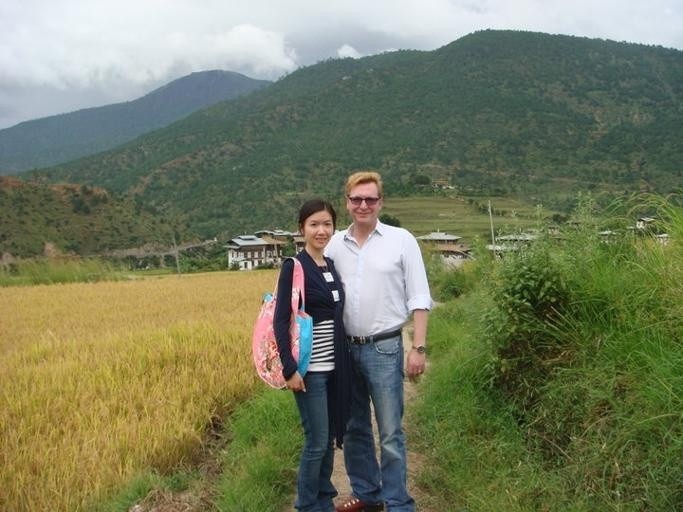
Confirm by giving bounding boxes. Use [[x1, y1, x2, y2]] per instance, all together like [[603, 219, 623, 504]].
[[347, 193, 381, 206]]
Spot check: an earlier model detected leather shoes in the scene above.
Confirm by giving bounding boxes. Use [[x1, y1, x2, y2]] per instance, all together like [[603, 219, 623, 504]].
[[336, 498, 384, 512]]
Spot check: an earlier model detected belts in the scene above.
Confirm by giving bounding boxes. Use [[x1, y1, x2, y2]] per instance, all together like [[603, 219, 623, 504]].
[[347, 329, 401, 345]]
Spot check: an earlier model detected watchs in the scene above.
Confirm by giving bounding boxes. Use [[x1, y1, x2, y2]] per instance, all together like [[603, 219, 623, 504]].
[[411, 344, 427, 353]]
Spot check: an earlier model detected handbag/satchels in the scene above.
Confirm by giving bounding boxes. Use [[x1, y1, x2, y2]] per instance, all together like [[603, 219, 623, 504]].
[[252, 294, 313, 390]]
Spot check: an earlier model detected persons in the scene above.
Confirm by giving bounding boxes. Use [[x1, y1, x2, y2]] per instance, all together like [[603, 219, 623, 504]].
[[324, 170, 433, 510], [274, 198, 351, 512]]
